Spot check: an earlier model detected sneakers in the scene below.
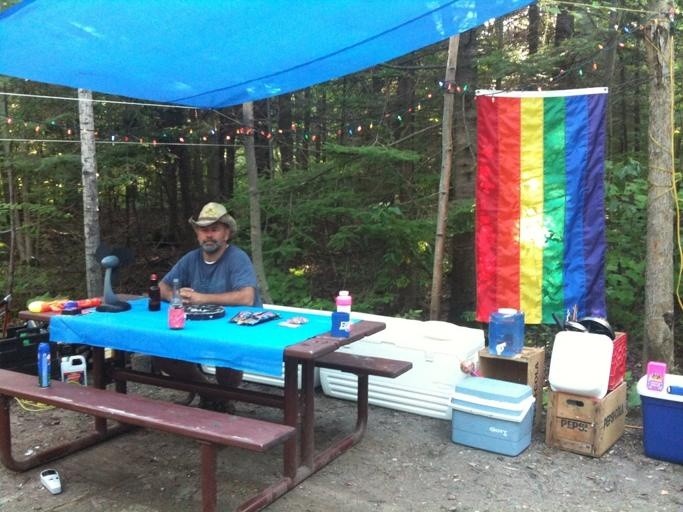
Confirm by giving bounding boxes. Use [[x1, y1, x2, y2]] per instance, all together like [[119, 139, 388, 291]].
[[199, 379, 236, 414]]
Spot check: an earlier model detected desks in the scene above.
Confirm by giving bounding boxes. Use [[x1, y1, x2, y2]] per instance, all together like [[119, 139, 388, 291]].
[[17, 293, 386, 512]]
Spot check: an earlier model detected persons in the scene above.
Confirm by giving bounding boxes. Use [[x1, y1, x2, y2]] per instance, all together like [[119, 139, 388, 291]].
[[156, 202, 263, 414]]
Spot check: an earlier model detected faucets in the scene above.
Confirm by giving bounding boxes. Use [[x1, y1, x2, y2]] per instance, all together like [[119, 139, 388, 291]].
[[495, 343, 508, 355]]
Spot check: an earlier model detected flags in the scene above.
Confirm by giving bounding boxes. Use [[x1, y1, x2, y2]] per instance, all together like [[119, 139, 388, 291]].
[[475, 86, 608, 325]]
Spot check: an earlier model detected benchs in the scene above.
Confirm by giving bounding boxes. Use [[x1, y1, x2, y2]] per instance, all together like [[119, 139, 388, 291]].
[[0, 364, 296, 511], [200, 303, 334, 391], [312, 353, 413, 475], [319, 312, 485, 421]]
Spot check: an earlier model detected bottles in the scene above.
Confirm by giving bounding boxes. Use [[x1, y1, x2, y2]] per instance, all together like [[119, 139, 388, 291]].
[[335, 296, 352, 312], [148, 274, 160, 311], [38, 342, 51, 390], [167, 278, 185, 329]]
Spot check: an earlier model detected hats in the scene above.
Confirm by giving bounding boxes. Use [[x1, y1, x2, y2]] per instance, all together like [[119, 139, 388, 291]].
[[187, 202, 237, 233]]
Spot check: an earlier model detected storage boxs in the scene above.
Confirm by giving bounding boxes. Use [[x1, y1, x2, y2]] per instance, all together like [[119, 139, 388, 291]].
[[548, 330, 613, 399], [636, 373, 683, 466], [447, 376, 536, 457], [608, 331, 627, 391], [545, 382, 627, 458], [479, 345, 545, 443]]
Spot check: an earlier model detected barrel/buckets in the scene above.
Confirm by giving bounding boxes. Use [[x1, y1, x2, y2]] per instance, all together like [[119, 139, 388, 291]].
[[488, 308, 525, 358], [59, 354, 89, 387]]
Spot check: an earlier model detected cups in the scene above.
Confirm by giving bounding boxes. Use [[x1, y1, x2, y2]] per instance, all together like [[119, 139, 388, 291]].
[[331, 312, 350, 339]]
